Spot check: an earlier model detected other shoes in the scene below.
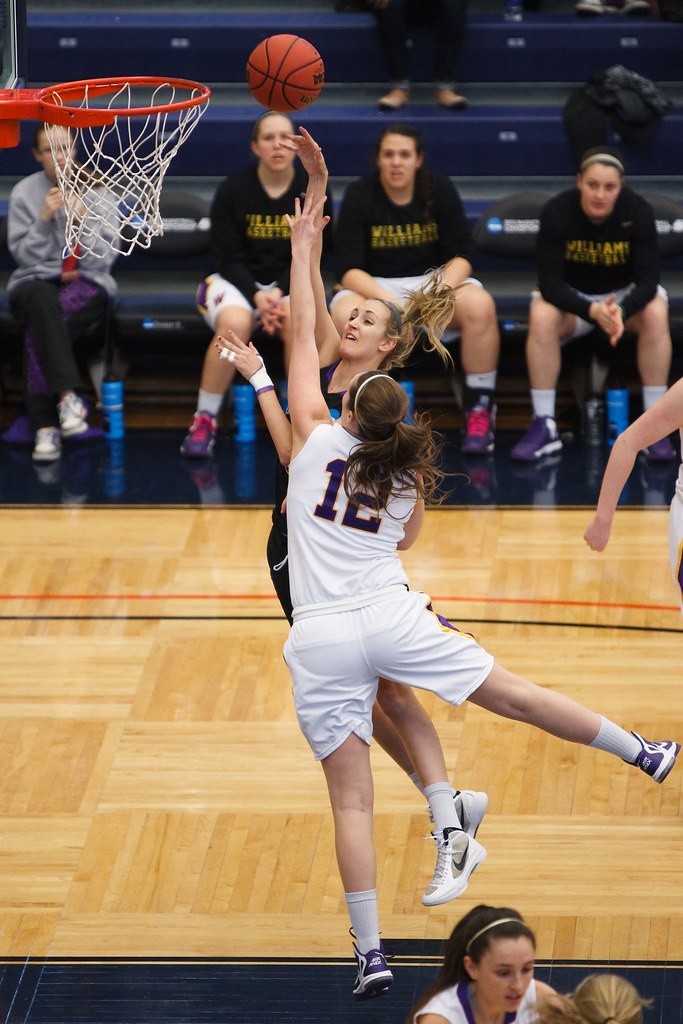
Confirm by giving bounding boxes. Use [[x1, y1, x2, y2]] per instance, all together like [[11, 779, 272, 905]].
[[438, 90, 467, 106], [377, 92, 410, 107], [504, 6, 523, 21], [575, 0, 604, 14], [622, 0, 650, 13]]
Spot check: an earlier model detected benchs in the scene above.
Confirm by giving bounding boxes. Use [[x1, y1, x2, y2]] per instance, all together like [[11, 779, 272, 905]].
[[0, 0, 683, 347]]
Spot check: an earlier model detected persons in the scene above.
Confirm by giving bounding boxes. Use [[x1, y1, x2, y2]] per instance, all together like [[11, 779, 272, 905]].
[[408, 906, 644, 1024], [279, 192, 681, 999], [6, 122, 121, 462], [330, 125, 500, 455], [177, 111, 332, 459], [215, 125, 484, 905], [371, 0, 469, 111], [508, 148, 677, 461], [585, 376, 683, 594]]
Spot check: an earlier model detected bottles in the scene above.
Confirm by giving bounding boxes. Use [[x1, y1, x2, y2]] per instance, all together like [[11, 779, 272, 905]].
[[399, 382, 414, 423], [52, 206, 67, 248], [608, 390, 629, 447], [585, 394, 605, 447], [280, 380, 289, 415], [100, 381, 124, 439], [327, 409, 339, 420], [233, 384, 256, 440]]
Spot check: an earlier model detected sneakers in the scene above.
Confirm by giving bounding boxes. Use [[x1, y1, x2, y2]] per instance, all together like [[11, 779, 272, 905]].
[[350, 926, 396, 1000], [180, 411, 218, 456], [57, 395, 88, 438], [425, 790, 488, 840], [32, 426, 63, 460], [642, 463, 671, 493], [421, 826, 488, 905], [514, 455, 560, 490], [34, 462, 60, 483], [193, 459, 218, 489], [466, 457, 497, 499], [642, 435, 676, 460], [60, 487, 88, 503], [510, 416, 564, 459], [461, 403, 497, 454], [622, 731, 680, 783]]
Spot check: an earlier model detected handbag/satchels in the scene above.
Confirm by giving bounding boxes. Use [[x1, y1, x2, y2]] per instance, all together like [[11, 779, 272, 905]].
[[563, 64, 672, 163]]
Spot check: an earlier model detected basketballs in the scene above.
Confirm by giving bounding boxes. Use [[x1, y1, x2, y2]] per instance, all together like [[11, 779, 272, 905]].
[[246, 33, 324, 113]]
